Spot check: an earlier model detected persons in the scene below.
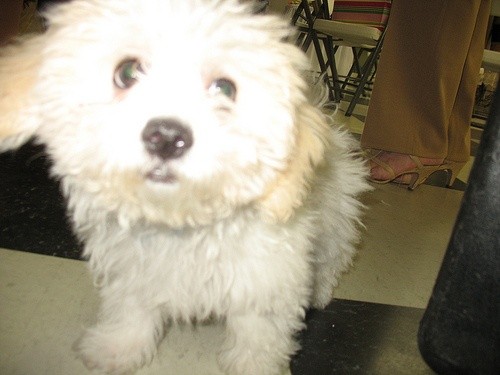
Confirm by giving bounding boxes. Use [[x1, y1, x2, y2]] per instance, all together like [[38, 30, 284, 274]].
[[360, 0, 491, 192]]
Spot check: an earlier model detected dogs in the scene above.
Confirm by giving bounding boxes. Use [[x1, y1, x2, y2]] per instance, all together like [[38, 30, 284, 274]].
[[0, 0, 378, 375]]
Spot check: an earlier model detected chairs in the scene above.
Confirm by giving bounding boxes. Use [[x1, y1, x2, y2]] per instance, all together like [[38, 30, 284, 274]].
[[280, 0, 392, 117]]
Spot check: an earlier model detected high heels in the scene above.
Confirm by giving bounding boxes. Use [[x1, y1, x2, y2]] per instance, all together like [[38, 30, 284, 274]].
[[392, 161, 466, 189], [365, 150, 441, 191]]
